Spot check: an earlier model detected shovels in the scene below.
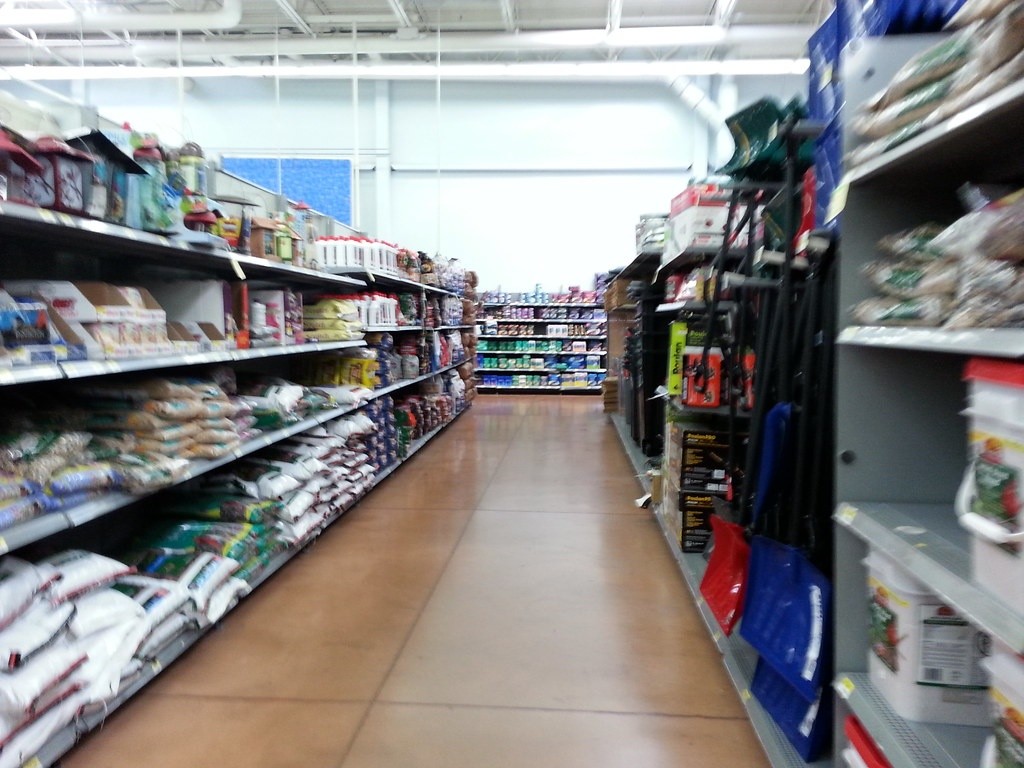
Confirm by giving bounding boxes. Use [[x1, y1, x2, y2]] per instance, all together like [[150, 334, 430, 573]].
[[696, 226, 840, 764], [691, 96, 837, 392]]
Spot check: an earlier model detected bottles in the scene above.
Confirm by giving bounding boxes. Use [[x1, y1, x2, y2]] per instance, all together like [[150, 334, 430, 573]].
[[249, 300, 266, 327]]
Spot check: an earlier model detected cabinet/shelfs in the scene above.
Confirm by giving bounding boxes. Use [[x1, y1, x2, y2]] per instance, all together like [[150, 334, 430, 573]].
[[837, 27, 1024, 768], [0, 72, 606, 768]]
